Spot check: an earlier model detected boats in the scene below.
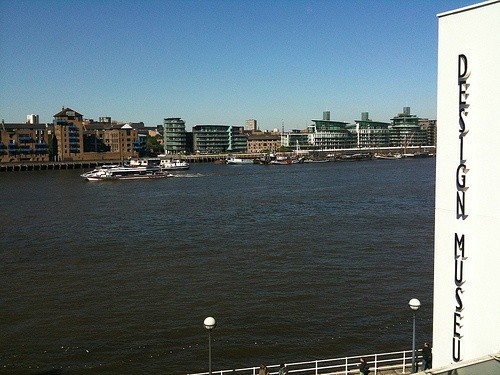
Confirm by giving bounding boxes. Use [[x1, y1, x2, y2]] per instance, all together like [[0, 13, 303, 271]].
[[225, 152, 436, 168], [101, 157, 190, 171], [79, 165, 173, 181]]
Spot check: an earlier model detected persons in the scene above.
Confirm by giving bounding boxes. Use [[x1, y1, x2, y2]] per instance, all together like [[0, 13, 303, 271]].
[[357, 357, 369, 375], [278, 362, 288, 375], [258, 363, 269, 375], [421, 342, 432, 371]]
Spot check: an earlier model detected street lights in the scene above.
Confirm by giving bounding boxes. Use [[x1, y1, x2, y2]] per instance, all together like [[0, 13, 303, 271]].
[[408, 298, 421, 373], [203, 317, 215, 375]]
